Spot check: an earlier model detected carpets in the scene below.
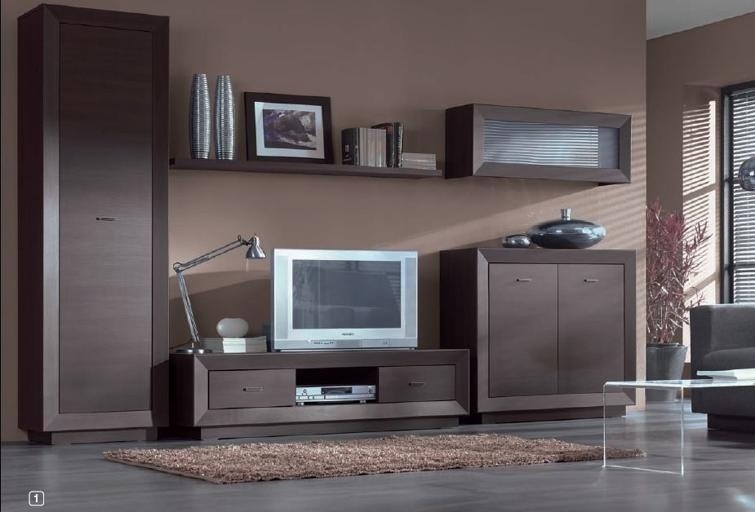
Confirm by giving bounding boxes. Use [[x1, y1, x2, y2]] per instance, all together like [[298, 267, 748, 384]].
[[101, 432, 648, 485]]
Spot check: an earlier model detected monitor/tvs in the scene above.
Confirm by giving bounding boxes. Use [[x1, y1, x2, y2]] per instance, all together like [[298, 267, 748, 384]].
[[271, 248, 419, 353]]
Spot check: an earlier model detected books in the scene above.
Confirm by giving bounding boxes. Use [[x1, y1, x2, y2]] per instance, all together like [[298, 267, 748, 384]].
[[339, 120, 438, 169], [696, 367, 755, 377], [198, 334, 267, 354], [711, 374, 755, 382]]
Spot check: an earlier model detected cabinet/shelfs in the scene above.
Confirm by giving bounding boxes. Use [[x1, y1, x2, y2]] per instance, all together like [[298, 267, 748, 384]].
[[171, 349, 470, 441], [439, 248, 636, 425], [19, 3, 169, 446]]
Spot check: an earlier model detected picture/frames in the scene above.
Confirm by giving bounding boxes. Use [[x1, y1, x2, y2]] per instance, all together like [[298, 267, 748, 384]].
[[244, 91, 334, 164]]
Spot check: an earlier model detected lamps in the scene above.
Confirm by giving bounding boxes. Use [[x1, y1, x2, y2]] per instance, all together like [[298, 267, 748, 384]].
[[173, 233, 268, 354]]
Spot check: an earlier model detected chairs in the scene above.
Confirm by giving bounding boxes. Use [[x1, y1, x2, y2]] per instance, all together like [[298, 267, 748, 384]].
[[691, 302, 755, 445]]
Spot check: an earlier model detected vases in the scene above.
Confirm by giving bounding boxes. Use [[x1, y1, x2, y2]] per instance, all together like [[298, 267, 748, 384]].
[[215, 74, 234, 160], [526, 207, 606, 249], [189, 73, 211, 159]]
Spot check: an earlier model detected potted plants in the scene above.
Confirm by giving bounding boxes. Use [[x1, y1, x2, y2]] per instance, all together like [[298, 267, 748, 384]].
[[646, 197, 714, 402]]
